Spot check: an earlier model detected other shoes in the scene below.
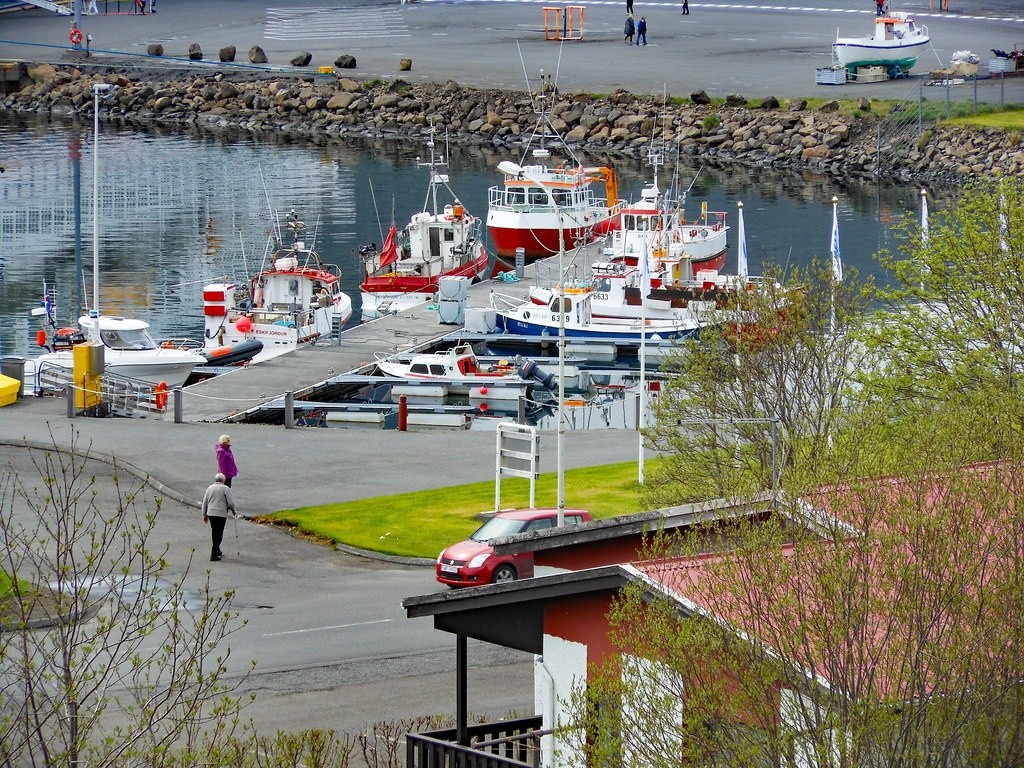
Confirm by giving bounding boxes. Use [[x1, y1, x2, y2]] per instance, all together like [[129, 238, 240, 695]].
[[685, 13, 690, 15], [631, 14, 634, 16], [210, 555, 221, 561], [681, 13, 685, 15], [636, 43, 640, 46], [642, 43, 647, 45], [624, 38, 626, 43], [625, 13, 630, 15], [629, 42, 634, 46], [217, 550, 223, 556]]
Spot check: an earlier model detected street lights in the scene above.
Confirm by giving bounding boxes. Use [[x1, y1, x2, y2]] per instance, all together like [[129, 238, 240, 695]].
[[86, 81, 116, 318], [497, 159, 566, 527]]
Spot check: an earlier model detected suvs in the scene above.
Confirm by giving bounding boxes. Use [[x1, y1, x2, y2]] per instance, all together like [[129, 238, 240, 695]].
[[432, 506, 593, 590]]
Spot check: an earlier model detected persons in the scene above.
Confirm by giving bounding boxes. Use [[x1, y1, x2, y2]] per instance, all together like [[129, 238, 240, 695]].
[[681, 0, 689, 15], [214, 434, 238, 488], [141, 0, 156, 13], [626, 0, 633, 14], [636, 16, 648, 45], [624, 13, 635, 44], [202, 473, 236, 561], [876, 0, 884, 15]]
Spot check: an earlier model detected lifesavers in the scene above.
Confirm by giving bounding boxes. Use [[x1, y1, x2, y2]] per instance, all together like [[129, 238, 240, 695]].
[[156, 381, 168, 409], [444, 214, 464, 221]]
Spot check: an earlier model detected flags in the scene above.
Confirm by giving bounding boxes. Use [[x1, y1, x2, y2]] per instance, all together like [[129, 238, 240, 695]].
[[636, 241, 651, 299], [742, 219, 748, 278], [45, 284, 51, 314], [831, 220, 843, 281]]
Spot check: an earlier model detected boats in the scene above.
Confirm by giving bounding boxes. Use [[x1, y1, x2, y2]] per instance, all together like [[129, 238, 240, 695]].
[[373, 331, 558, 401], [356, 116, 492, 324], [829, 1, 932, 78], [484, 80, 796, 355], [199, 163, 354, 364], [186, 336, 264, 367], [19, 270, 207, 402], [484, 37, 630, 260]]
